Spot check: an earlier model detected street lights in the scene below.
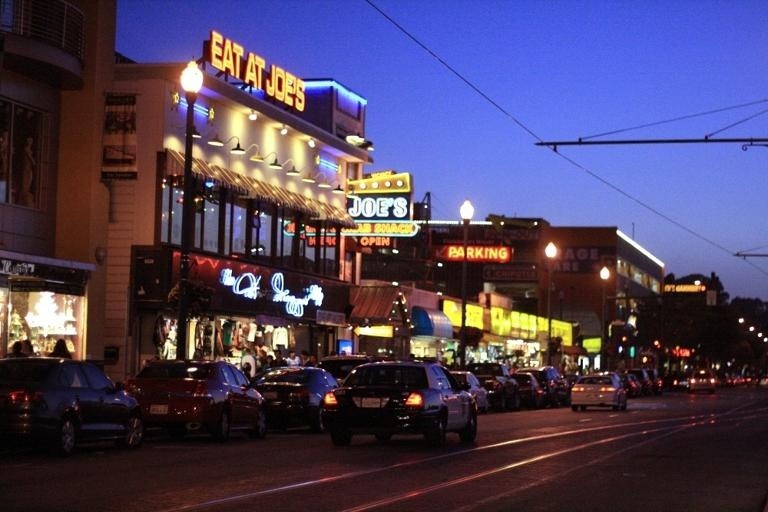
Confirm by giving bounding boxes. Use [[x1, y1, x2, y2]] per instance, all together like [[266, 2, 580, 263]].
[[596, 265, 611, 367], [544, 242, 561, 361], [457, 198, 474, 369], [175, 60, 204, 361]]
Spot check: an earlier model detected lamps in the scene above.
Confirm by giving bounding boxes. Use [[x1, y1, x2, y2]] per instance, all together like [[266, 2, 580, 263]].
[[173, 118, 346, 194]]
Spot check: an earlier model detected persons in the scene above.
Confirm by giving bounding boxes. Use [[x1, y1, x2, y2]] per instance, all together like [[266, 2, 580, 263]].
[[265, 349, 477, 368], [44, 337, 78, 360], [3, 341, 29, 357], [17, 132, 37, 206], [0, 126, 12, 204], [22, 339, 36, 357]]
